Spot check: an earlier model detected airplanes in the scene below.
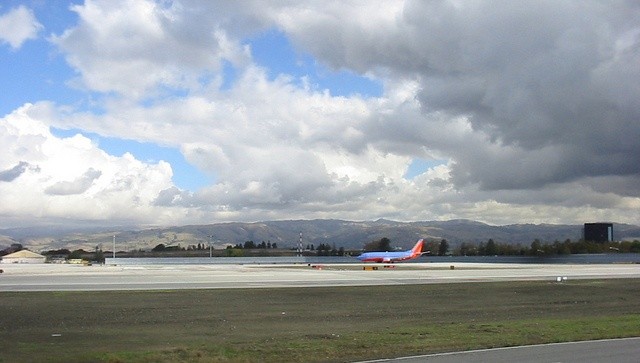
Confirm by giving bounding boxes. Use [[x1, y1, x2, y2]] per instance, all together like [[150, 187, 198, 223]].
[[356, 238, 431, 263]]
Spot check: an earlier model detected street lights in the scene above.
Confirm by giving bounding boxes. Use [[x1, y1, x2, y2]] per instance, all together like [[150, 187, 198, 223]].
[[207, 233, 216, 257]]
